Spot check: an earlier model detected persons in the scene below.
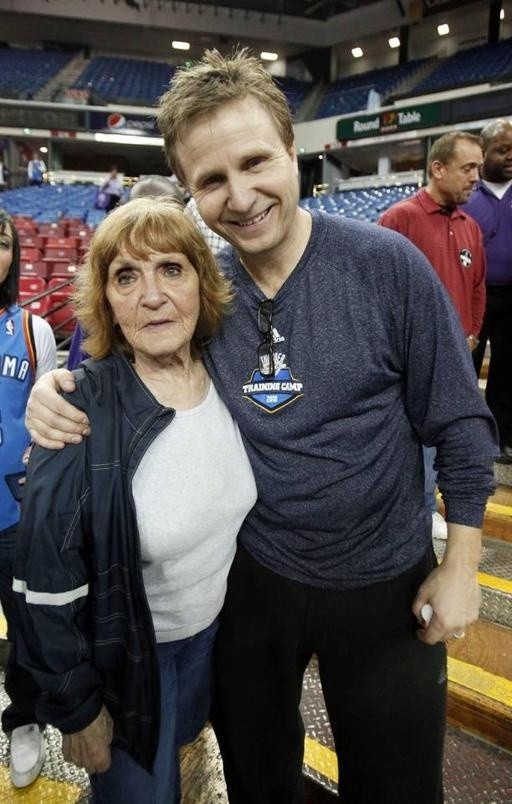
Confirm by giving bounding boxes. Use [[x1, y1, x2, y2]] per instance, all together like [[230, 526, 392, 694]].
[[378, 129, 487, 542], [66, 175, 184, 371], [98, 164, 125, 216], [0, 210, 59, 790], [22, 47, 499, 803], [185, 197, 232, 256], [28, 151, 47, 185], [459, 122, 512, 463], [9, 196, 260, 802]]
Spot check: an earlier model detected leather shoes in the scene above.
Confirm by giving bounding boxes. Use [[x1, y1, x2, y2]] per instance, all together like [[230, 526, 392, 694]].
[[10, 724, 45, 788]]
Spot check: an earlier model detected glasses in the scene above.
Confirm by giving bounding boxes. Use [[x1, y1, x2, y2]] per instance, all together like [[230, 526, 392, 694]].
[[251, 299, 274, 377]]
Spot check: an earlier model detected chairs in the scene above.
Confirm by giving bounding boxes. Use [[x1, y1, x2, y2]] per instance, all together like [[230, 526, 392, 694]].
[[255, 180, 430, 234], [0, 163, 147, 362]]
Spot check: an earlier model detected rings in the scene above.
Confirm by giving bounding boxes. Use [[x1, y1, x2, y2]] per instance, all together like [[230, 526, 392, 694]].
[[453, 631, 465, 639]]
